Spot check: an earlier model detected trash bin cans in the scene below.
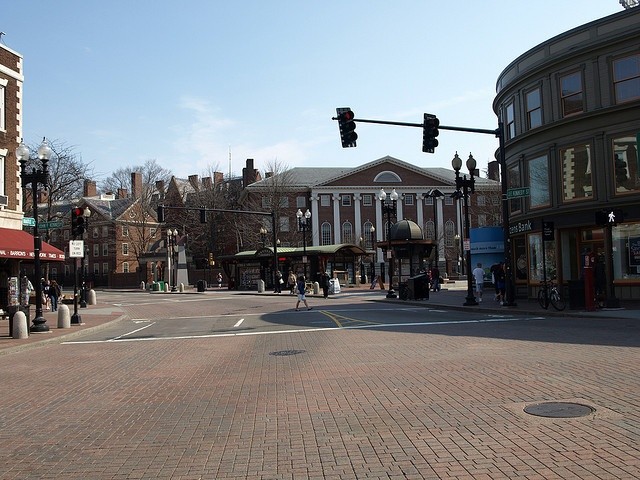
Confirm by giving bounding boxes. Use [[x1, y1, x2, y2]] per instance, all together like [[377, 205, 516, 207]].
[[398, 282, 408, 300]]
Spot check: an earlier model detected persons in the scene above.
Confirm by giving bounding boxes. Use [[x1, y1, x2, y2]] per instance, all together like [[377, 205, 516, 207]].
[[22, 277, 62, 313], [287, 271, 297, 294], [272, 271, 284, 294], [490, 263, 507, 304], [321, 271, 330, 299], [429, 267, 441, 293], [473, 264, 485, 303], [294, 276, 313, 312]]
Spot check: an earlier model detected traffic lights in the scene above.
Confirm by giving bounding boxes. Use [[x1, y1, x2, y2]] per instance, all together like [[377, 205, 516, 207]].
[[72, 206, 84, 236], [423, 113, 439, 154], [336, 106, 357, 148]]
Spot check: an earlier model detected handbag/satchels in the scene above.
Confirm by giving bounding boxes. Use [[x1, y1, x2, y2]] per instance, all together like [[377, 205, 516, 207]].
[[279, 278, 284, 285]]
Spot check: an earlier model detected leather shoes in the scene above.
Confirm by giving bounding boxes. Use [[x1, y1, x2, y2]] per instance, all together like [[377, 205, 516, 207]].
[[308, 308, 312, 311], [295, 309, 300, 311]]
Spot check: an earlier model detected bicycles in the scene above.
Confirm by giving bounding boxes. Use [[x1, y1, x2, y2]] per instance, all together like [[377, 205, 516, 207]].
[[537, 275, 569, 311]]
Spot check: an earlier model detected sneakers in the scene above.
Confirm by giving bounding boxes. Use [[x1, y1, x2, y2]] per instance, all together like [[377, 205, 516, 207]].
[[479, 297, 483, 303]]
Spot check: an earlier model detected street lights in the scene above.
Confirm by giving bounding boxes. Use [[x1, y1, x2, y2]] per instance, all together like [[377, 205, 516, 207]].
[[15, 137, 53, 331], [451, 151, 478, 305], [260, 227, 268, 283], [296, 209, 311, 282], [166, 228, 178, 291], [378, 188, 399, 298], [370, 225, 377, 281]]
